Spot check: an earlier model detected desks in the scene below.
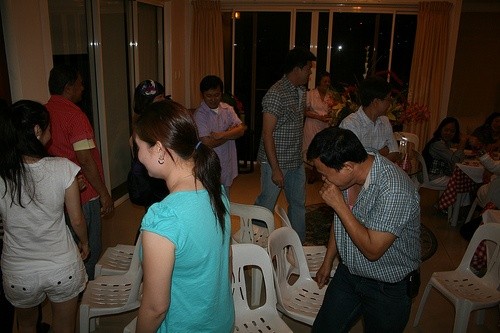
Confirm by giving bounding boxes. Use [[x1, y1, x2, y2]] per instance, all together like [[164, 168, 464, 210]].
[[446, 147, 500, 225], [466, 204, 500, 275]]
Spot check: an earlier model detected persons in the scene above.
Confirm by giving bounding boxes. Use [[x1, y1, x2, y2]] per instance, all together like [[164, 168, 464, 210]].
[[0, 100, 91, 333], [251, 46, 317, 245], [40, 63, 115, 301], [133, 96, 234, 333], [192, 75, 245, 200], [127, 80, 171, 206], [306, 125, 423, 333], [302, 73, 500, 240]]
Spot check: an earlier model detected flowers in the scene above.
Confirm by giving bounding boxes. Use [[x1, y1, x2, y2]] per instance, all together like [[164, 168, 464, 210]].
[[326, 78, 432, 126]]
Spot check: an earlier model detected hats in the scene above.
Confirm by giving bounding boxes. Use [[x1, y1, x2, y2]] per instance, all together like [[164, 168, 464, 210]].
[[132, 80, 164, 114]]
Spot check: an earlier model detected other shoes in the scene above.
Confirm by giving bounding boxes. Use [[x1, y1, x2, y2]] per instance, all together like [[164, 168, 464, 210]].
[[308, 175, 316, 184]]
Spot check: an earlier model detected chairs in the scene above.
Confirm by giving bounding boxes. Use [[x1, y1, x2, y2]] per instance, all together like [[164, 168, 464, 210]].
[[79, 199, 338, 333], [388, 131, 453, 222], [414, 225, 500, 333]]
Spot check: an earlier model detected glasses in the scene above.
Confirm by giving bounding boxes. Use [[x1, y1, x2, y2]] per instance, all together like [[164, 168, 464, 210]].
[[382, 96, 392, 105]]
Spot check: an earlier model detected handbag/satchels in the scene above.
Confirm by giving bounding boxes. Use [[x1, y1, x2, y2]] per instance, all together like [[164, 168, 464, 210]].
[[127, 160, 170, 206]]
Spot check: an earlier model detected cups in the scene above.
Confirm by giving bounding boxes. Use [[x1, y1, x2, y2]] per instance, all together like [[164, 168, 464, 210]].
[[399, 139, 408, 154]]
